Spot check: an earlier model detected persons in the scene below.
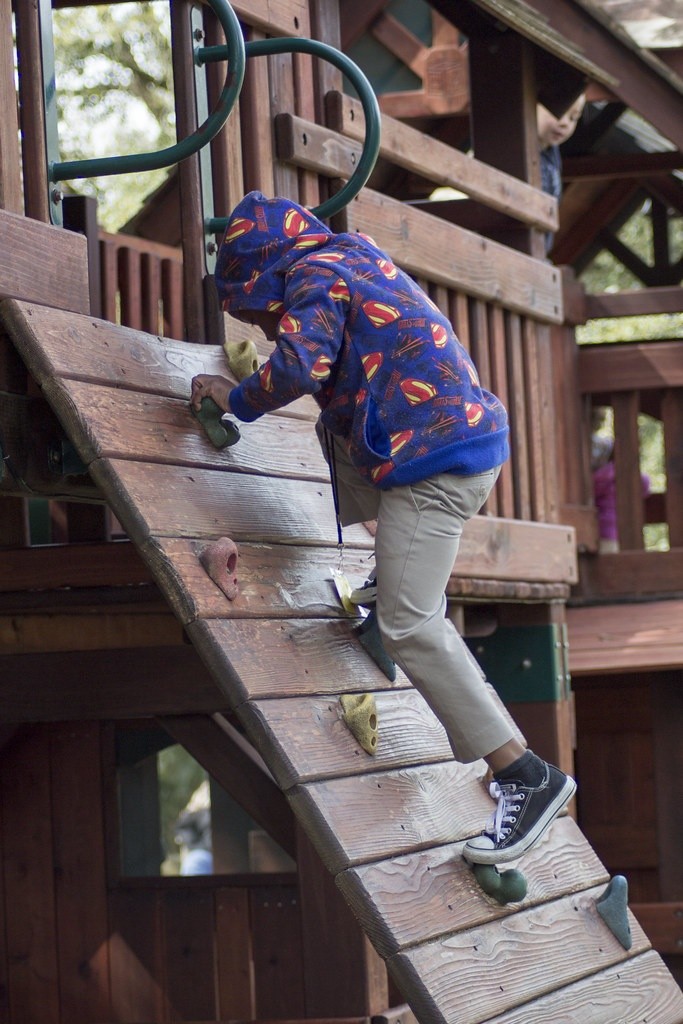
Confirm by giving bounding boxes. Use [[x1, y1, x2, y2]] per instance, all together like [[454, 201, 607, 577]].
[[590, 424, 654, 559], [174, 810, 216, 875], [185, 189, 582, 866], [535, 85, 589, 257]]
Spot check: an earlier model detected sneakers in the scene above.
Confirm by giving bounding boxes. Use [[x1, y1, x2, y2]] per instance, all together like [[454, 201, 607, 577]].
[[350, 578, 377, 604], [463, 760, 577, 864]]
